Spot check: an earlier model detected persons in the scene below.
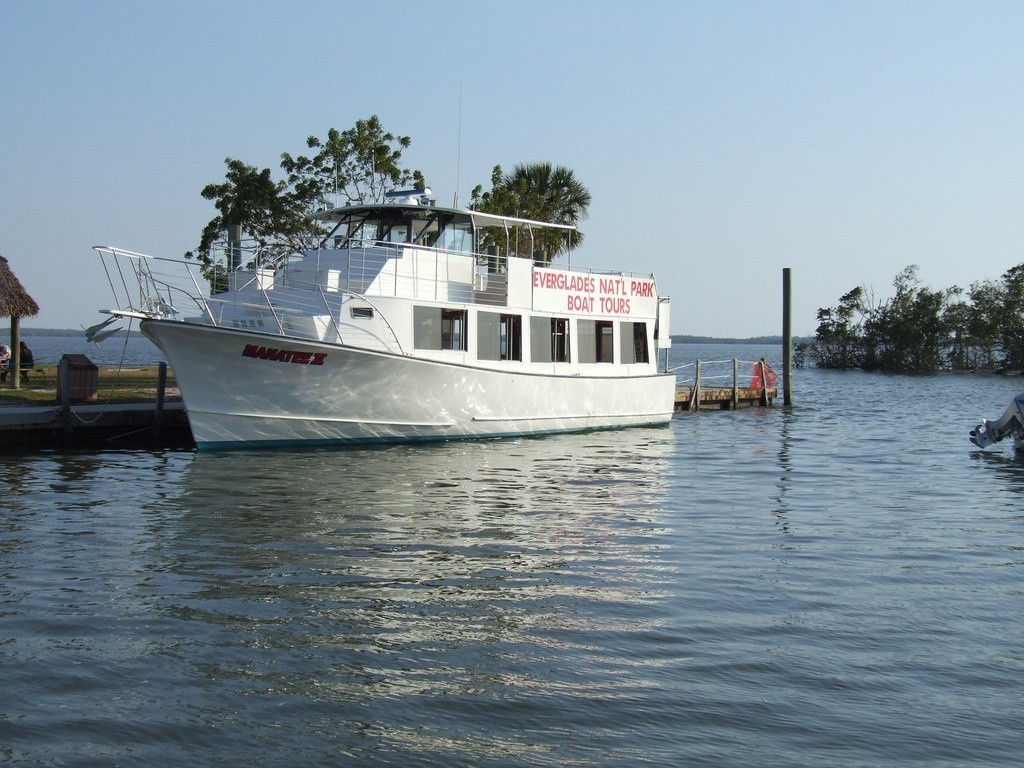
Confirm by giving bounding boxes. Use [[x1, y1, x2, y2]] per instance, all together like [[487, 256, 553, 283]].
[[20, 342, 34, 382], [0, 342, 11, 381]]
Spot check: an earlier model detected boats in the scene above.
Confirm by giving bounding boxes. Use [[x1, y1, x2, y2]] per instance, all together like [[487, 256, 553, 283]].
[[89, 150, 680, 457]]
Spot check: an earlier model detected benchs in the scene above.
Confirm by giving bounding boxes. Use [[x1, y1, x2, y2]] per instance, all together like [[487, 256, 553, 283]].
[[0, 369, 48, 383]]
